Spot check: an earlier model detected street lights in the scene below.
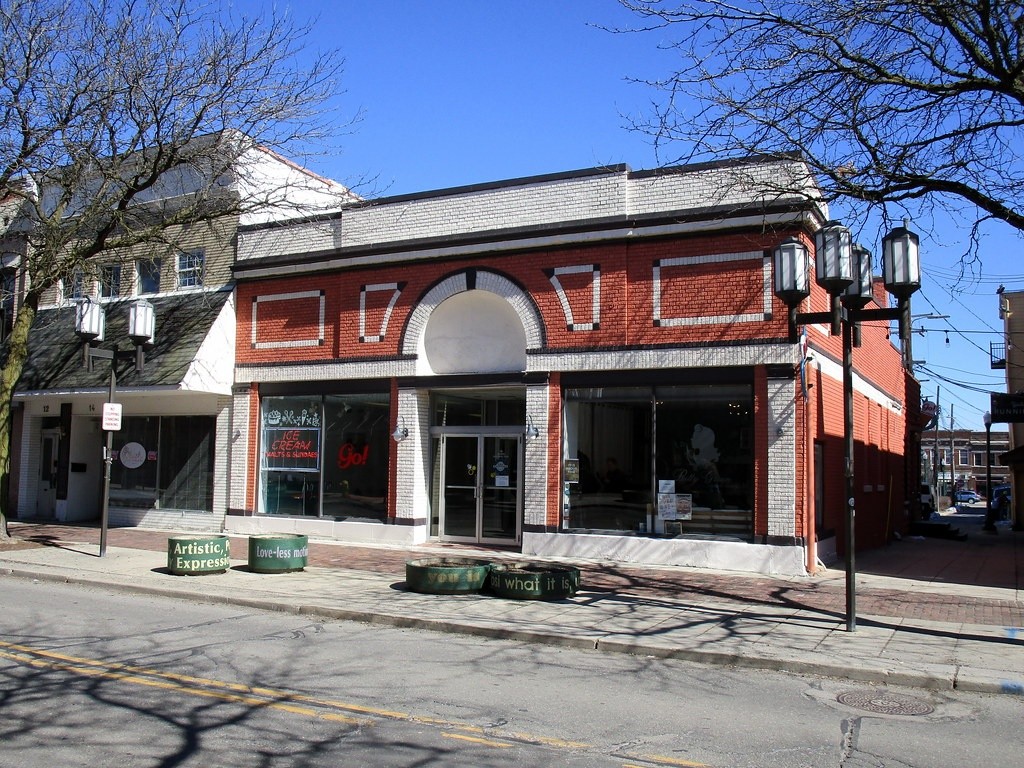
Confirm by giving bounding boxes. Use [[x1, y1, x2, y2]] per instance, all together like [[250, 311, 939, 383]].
[[923, 452, 928, 483], [72, 297, 158, 556], [941, 459, 946, 493], [983, 410, 997, 531], [772, 217, 920, 629]]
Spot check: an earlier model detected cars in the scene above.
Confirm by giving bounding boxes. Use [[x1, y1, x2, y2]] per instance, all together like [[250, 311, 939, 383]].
[[956, 491, 982, 503], [288, 490, 316, 502]]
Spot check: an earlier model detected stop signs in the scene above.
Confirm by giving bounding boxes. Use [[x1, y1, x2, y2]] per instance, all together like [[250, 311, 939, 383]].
[[956, 479, 965, 487]]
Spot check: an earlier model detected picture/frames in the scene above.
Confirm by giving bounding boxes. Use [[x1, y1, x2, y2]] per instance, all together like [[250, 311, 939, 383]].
[[740, 428, 749, 449]]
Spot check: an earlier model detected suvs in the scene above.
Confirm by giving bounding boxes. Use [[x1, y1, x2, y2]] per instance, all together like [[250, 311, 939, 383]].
[[992, 485, 1012, 504]]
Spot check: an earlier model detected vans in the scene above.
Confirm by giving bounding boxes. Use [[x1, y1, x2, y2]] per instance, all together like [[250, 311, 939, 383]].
[[921, 483, 934, 520]]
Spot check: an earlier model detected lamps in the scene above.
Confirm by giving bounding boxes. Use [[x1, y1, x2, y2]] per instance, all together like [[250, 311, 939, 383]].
[[526, 415, 541, 439], [392, 414, 408, 442]]
